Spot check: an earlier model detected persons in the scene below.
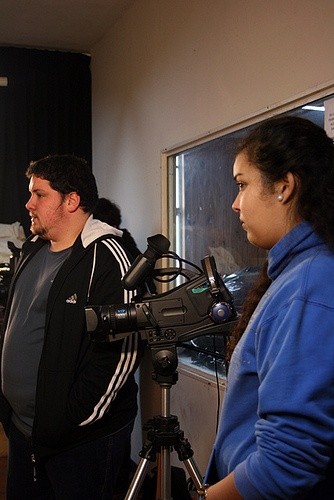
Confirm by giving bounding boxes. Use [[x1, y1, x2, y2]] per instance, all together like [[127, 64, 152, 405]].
[[0, 155, 148, 500], [195, 116, 334, 500]]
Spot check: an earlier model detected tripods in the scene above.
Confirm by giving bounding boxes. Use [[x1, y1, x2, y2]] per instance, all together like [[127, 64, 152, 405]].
[[124, 344, 205, 500]]
